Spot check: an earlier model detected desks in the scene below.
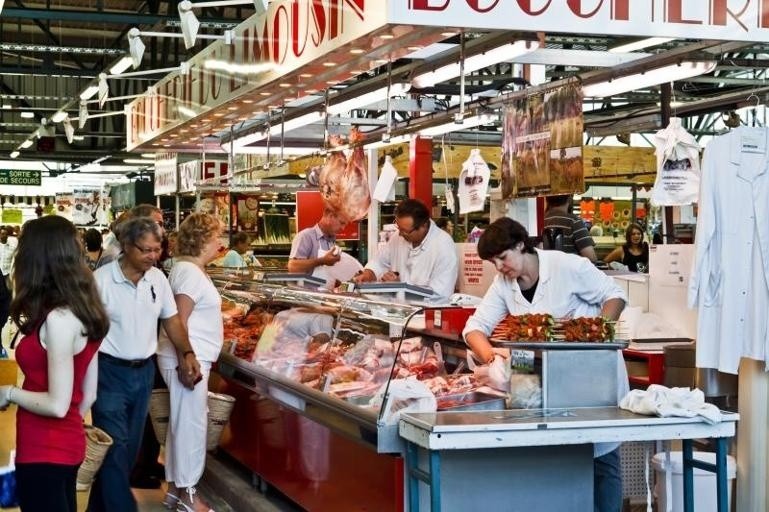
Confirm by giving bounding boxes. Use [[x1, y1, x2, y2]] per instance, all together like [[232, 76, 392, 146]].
[[398, 408, 740, 512]]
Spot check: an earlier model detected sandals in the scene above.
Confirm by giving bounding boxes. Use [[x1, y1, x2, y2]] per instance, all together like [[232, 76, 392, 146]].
[[160, 491, 179, 507], [176, 498, 216, 512]]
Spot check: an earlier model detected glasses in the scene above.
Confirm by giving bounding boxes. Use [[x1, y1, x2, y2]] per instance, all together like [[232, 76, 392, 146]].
[[133, 241, 162, 254], [392, 219, 415, 239]]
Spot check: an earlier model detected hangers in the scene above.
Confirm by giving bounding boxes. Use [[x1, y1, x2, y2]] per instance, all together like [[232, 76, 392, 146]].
[[741, 95, 765, 152]]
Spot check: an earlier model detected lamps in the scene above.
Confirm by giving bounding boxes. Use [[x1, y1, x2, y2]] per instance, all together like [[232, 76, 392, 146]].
[[63, 0, 272, 143]]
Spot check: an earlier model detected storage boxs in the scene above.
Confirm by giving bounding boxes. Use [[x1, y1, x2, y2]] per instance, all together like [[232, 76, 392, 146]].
[[425, 306, 476, 332], [612, 243, 695, 316]]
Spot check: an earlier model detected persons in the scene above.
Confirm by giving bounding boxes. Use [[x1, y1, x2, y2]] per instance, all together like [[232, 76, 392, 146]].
[[90, 216, 201, 511], [352, 198, 460, 300], [288, 207, 352, 284], [1, 203, 168, 332], [251, 300, 340, 414], [543, 193, 597, 262], [154, 210, 225, 512], [460, 218, 629, 512], [1, 215, 110, 511], [220, 231, 249, 267], [601, 223, 650, 273]]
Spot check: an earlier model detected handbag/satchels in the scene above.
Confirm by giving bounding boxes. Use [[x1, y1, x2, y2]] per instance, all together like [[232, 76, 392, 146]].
[[75, 424, 115, 492], [148, 388, 236, 452]]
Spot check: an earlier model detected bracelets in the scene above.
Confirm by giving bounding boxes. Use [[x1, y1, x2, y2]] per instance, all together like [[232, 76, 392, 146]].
[[182, 350, 195, 357], [5, 383, 17, 403]]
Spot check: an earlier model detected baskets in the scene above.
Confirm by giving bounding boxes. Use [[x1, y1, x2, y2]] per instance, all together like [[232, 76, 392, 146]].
[[619, 441, 656, 501]]
